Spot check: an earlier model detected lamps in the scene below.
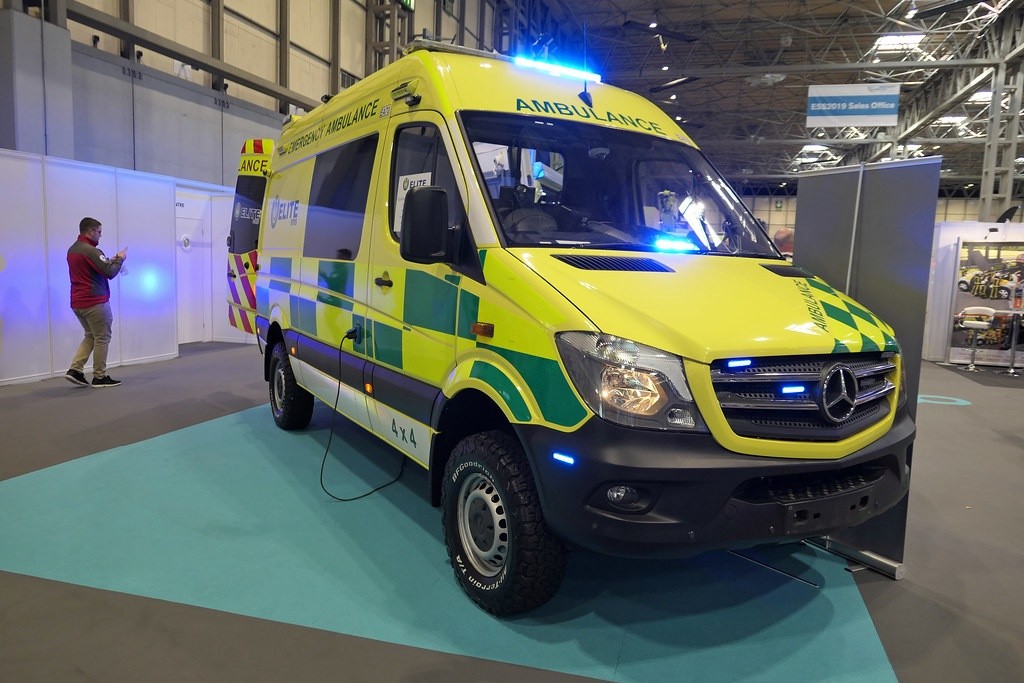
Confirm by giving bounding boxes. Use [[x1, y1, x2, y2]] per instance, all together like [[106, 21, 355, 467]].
[[531, 32, 555, 62]]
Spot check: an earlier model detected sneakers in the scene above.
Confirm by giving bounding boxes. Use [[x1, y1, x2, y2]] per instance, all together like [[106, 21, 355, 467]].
[[65, 369, 89, 386], [91, 375, 121, 387]]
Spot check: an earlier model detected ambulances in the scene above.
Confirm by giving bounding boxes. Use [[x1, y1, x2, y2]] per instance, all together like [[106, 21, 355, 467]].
[[227, 36, 918, 620]]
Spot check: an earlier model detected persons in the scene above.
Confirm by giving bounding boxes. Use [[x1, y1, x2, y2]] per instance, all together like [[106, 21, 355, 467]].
[[66, 217, 128, 387]]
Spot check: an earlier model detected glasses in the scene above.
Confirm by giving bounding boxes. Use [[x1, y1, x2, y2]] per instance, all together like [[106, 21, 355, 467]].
[[92, 229, 101, 235]]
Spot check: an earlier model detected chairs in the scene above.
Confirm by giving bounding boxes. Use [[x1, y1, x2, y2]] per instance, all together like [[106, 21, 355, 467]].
[[550, 160, 624, 231]]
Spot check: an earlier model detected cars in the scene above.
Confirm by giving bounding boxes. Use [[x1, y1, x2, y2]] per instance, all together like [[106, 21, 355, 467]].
[[953, 307, 1024, 350], [958, 264, 1024, 299]]
